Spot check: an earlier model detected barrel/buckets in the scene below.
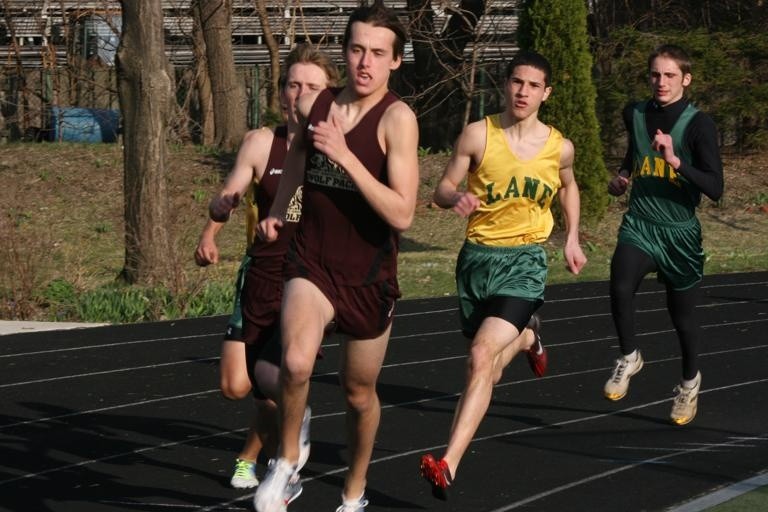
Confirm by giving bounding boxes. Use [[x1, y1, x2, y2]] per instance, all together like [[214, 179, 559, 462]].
[[53, 105, 121, 143]]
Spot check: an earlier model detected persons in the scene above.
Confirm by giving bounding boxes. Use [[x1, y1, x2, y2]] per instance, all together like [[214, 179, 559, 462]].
[[195, 1, 421, 512], [416, 48, 587, 499], [602, 44, 725, 426]]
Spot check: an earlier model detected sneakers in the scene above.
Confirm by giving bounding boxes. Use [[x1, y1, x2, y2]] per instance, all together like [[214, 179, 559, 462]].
[[670, 370, 702, 426], [527, 314, 547, 377], [231, 459, 260, 488], [420, 453, 452, 502], [603, 348, 644, 401], [335, 490, 365, 512], [253, 405, 311, 512]]
